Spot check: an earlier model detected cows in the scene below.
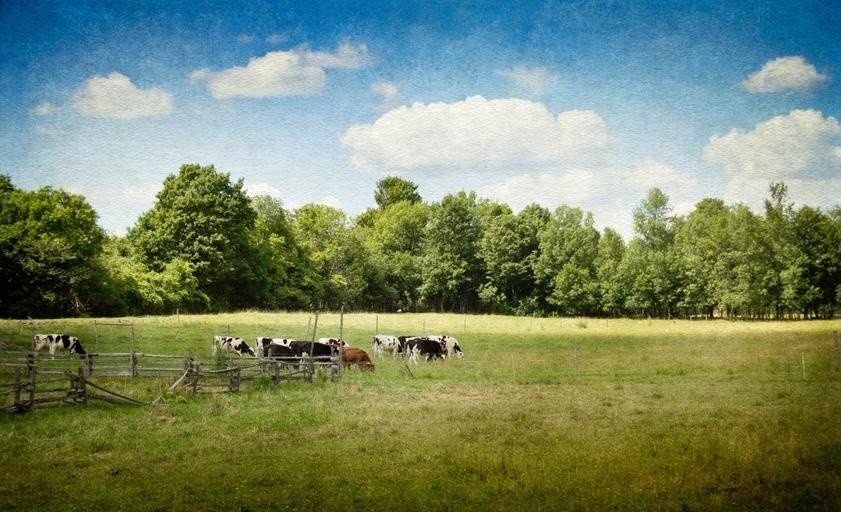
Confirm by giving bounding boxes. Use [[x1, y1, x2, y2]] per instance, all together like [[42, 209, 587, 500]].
[[370, 334, 465, 366], [31, 333, 87, 360], [210, 335, 376, 375]]
